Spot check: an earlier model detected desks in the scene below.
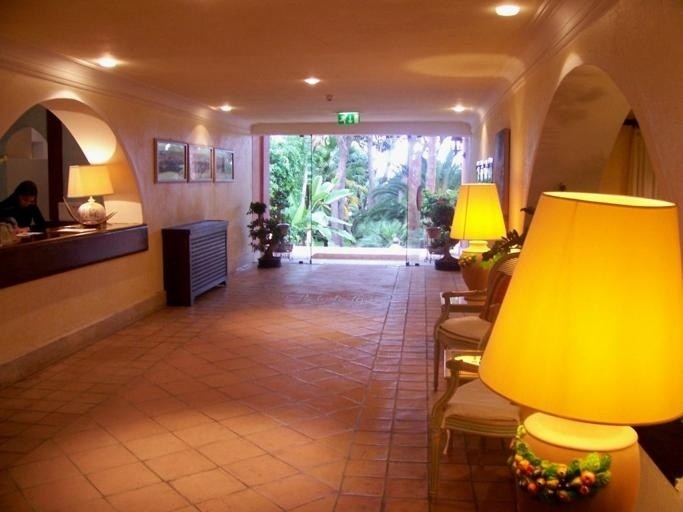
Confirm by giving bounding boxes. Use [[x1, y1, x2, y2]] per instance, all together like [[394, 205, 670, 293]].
[[0, 223, 149, 291], [443, 350, 482, 457], [439, 290, 484, 319]]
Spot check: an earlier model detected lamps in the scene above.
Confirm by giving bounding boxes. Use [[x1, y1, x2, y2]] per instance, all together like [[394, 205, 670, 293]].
[[478, 191, 683, 450], [67, 166, 115, 221], [450, 183, 507, 254]]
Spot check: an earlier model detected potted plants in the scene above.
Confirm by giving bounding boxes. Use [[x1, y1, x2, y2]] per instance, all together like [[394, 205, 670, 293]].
[[425, 204, 460, 270], [269, 186, 294, 252], [420, 190, 451, 255], [246, 201, 289, 268]]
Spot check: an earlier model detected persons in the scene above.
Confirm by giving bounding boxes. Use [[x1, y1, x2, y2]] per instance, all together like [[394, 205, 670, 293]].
[[0, 180, 47, 233]]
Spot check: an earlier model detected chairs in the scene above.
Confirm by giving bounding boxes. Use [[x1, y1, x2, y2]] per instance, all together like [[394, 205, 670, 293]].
[[434, 253, 520, 391], [427, 351, 523, 512]]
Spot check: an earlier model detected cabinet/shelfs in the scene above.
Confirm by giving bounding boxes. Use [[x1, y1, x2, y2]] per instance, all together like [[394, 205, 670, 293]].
[[161, 219, 228, 307]]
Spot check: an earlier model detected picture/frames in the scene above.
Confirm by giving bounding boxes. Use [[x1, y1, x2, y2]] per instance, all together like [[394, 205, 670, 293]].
[[154, 138, 188, 184], [214, 148, 236, 183], [188, 143, 214, 183]]
